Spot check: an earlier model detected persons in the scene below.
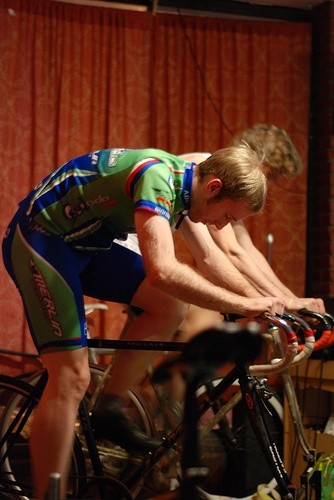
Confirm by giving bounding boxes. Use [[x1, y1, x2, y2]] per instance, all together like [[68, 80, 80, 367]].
[[0, 121, 326, 500]]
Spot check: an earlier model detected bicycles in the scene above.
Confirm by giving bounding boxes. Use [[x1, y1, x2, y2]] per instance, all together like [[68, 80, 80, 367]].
[[0, 304, 334, 500]]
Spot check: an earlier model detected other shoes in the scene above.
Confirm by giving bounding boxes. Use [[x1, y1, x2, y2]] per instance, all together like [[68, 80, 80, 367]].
[[81, 408, 160, 455]]
